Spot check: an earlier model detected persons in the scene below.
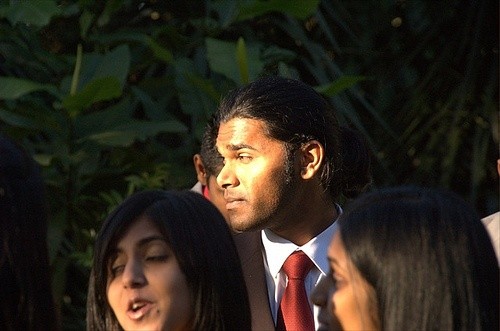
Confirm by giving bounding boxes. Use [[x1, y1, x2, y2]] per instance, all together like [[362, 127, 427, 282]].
[[190, 74, 344, 331], [480, 157, 500, 266], [85, 188, 252, 331], [309, 185, 500, 331]]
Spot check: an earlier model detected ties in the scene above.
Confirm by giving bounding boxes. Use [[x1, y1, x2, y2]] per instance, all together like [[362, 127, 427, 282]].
[[277, 251, 314, 331]]
